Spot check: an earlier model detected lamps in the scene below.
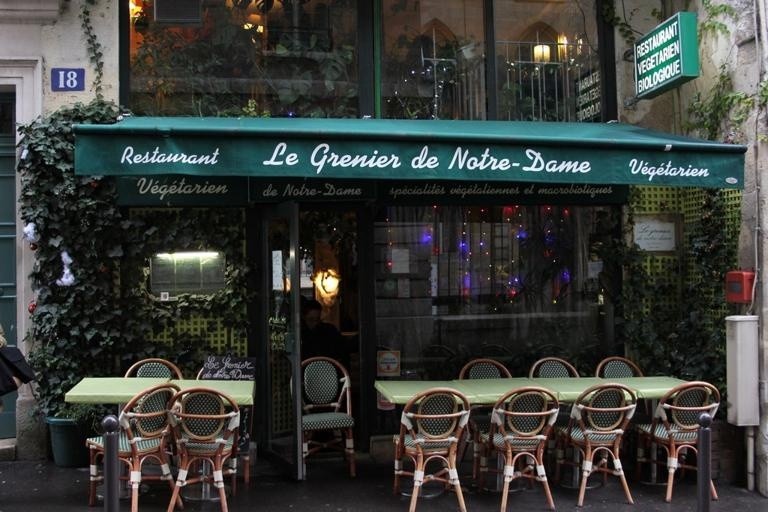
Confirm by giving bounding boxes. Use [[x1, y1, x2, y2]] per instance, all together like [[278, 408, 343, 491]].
[[322, 267, 343, 293], [242, 22, 264, 45], [534, 45, 550, 63]]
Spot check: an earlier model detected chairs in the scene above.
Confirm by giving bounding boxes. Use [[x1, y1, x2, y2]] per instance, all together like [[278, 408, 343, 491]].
[[596, 356, 649, 461], [196, 366, 250, 487], [288, 356, 356, 481], [391, 387, 470, 512], [478, 385, 560, 512], [165, 387, 241, 512], [632, 380, 721, 504], [85, 383, 183, 512], [459, 358, 513, 462], [528, 356, 580, 454], [551, 383, 639, 508], [419, 344, 578, 365], [118, 358, 183, 486]]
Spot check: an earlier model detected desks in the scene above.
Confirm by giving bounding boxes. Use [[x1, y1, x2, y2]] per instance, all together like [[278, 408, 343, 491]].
[[168, 378, 255, 504], [532, 377, 628, 490], [373, 380, 477, 503], [65, 376, 170, 500], [603, 375, 712, 487], [453, 377, 557, 496]]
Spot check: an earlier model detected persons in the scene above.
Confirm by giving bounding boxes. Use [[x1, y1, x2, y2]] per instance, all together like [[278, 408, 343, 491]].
[[0, 323, 37, 398], [299, 298, 350, 455]]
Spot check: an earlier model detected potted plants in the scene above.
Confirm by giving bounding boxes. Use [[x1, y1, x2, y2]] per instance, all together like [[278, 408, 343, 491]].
[[43, 401, 112, 469]]
